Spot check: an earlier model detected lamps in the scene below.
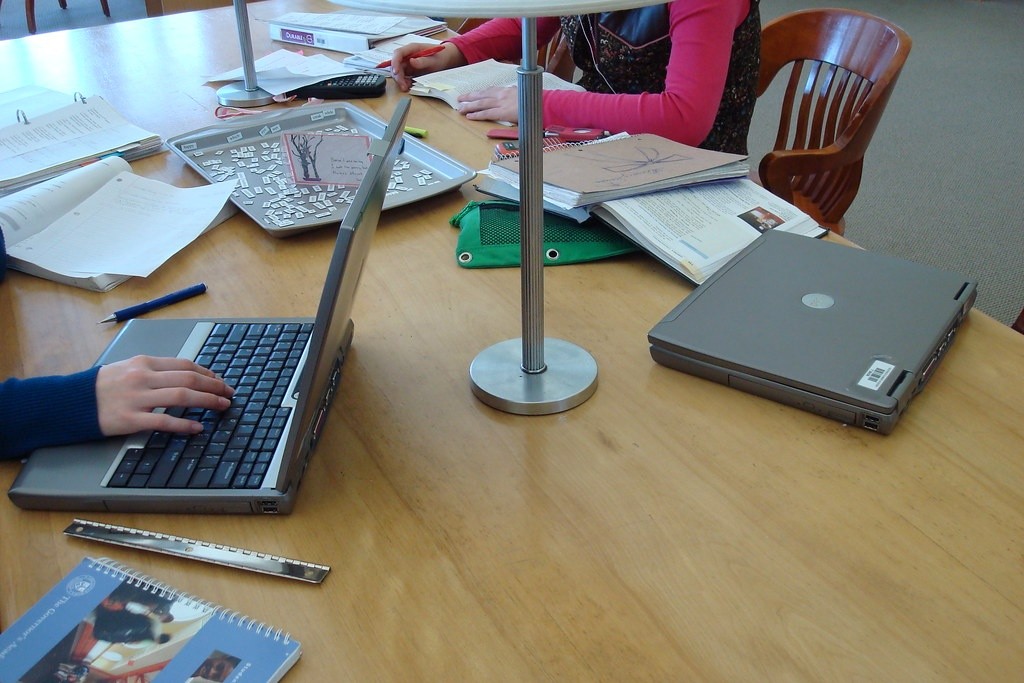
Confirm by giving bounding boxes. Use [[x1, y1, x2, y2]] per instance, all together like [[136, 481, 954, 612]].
[[328, 0, 679, 416]]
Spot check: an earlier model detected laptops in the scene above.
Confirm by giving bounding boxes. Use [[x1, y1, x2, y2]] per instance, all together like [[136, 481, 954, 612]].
[[648, 229, 979, 437], [6, 95, 416, 516]]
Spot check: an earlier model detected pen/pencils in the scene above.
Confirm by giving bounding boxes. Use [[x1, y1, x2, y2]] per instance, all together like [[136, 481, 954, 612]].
[[404, 125, 429, 138], [373, 46, 446, 70], [98, 283, 209, 325]]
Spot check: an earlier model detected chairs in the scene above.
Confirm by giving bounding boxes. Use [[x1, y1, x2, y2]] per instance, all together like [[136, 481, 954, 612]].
[[453, 17, 576, 84], [745, 9, 912, 239]]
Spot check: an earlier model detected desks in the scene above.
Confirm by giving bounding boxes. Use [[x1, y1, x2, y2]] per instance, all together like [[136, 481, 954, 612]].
[[0, 0, 1024, 683]]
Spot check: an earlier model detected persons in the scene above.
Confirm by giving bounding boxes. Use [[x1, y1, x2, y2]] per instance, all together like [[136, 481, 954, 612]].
[[390, 0, 762, 159], [0, 354, 235, 467]]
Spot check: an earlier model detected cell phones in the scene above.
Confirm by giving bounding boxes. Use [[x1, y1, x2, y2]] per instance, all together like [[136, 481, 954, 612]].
[[494, 128, 604, 160]]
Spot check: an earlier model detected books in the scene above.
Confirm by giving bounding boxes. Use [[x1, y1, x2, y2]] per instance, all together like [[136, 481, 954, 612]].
[[0, 91, 240, 292], [476, 132, 828, 286], [409, 58, 584, 126], [1, 558, 299, 681]]
